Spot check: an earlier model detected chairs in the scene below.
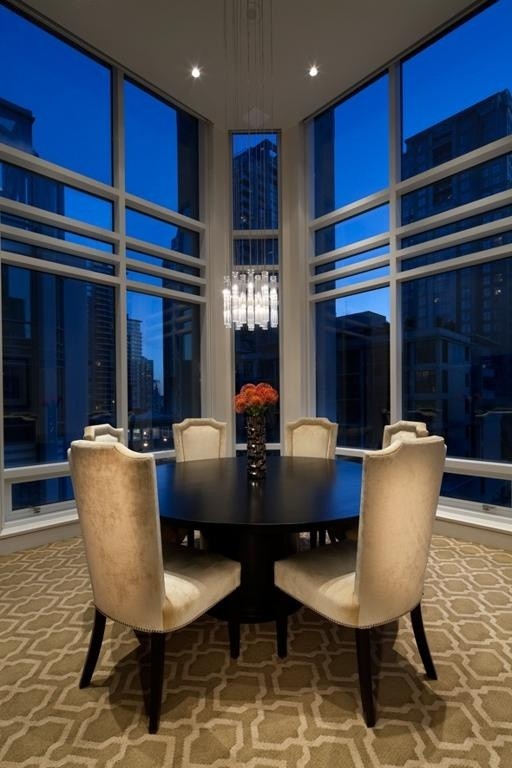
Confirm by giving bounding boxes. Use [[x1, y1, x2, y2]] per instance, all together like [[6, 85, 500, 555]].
[[175, 418, 227, 551], [84, 422, 187, 550], [285, 417, 338, 547], [275, 435, 448, 729], [68, 440, 242, 734], [328, 420, 429, 538]]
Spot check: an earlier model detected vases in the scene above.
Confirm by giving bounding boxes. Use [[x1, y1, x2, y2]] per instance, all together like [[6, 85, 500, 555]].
[[246, 420, 268, 482]]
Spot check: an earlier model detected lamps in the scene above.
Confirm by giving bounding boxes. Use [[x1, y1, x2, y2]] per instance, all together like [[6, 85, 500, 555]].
[[221, 0, 279, 332]]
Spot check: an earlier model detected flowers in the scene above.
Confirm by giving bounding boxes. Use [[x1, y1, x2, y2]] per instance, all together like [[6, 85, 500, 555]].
[[231, 383, 279, 422]]
